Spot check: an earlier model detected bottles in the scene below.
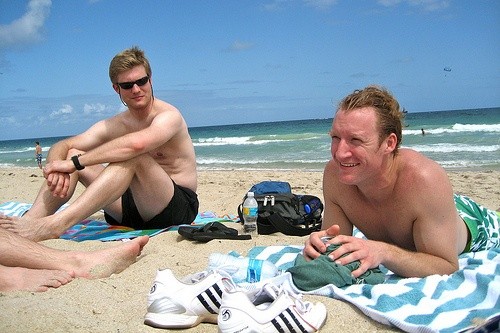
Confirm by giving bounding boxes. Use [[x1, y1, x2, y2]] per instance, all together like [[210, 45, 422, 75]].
[[243, 191, 258, 238], [208, 252, 286, 283], [304, 197, 321, 214]]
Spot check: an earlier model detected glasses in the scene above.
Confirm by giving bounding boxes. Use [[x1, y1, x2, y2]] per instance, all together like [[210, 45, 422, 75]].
[[115, 73, 149, 89]]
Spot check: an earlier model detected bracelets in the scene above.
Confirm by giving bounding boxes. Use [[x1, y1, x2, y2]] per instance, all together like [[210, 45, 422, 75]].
[[70, 153, 85, 170]]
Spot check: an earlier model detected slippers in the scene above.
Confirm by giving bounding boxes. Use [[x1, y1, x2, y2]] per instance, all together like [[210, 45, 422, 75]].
[[193, 224, 252, 241], [178, 221, 238, 238]]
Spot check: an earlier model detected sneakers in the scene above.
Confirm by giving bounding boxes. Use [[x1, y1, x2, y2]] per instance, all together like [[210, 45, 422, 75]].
[[144, 268, 240, 327], [217, 280, 328, 333]]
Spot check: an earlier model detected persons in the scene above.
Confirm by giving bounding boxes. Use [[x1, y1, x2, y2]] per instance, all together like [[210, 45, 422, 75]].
[[36, 141, 42, 169], [0, 227, 149, 292], [302, 84, 500, 279], [0, 45, 199, 243]]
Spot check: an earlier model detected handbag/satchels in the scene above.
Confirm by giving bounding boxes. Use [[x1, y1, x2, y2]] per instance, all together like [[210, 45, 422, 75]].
[[237, 180, 324, 236]]
